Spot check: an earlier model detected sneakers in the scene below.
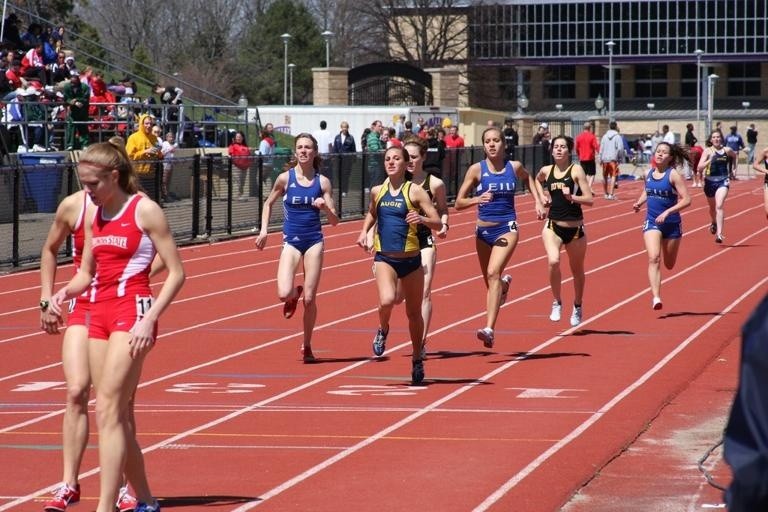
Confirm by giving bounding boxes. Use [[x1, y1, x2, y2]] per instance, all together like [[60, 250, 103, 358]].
[[570, 304, 582, 326], [301, 344, 314, 363], [604, 195, 608, 200], [364, 188, 370, 195], [716, 238, 722, 243], [710, 224, 716, 234], [116, 484, 136, 512], [608, 195, 616, 200], [134, 496, 160, 512], [698, 183, 703, 188], [477, 327, 494, 347], [33, 144, 46, 152], [284, 286, 303, 319], [44, 482, 80, 511], [500, 275, 512, 306], [550, 299, 562, 321], [653, 298, 662, 310], [373, 325, 389, 356], [17, 145, 34, 153], [692, 183, 697, 188], [342, 192, 347, 197], [412, 360, 424, 386]]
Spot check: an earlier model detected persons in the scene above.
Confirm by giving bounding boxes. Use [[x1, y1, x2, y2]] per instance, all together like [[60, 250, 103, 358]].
[[41, 189, 137, 512], [503, 116, 767, 347], [330, 114, 464, 186], [1, 10, 276, 203], [720, 295, 768, 511], [366, 137, 449, 360], [255, 133, 338, 362], [453, 127, 547, 348], [356, 145, 442, 384], [47, 135, 185, 512]]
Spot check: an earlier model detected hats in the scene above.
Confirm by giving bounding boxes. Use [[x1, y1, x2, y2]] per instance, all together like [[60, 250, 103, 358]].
[[584, 122, 592, 127], [16, 88, 29, 97], [26, 86, 41, 96], [125, 87, 133, 95], [540, 123, 548, 129]]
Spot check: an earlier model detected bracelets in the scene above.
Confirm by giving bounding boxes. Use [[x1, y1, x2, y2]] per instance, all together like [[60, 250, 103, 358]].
[[40, 300, 48, 309], [441, 223, 448, 228]]
